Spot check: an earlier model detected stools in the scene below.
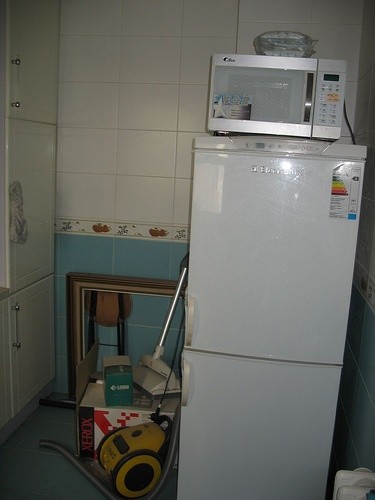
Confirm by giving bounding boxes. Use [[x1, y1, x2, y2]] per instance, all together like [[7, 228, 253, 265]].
[[84, 291, 133, 356]]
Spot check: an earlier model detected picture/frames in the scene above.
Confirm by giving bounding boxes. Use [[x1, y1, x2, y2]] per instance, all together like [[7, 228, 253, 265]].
[[66, 273, 187, 401]]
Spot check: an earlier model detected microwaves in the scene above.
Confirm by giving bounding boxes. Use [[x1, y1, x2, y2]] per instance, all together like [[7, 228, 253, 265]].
[[207, 53, 347, 141]]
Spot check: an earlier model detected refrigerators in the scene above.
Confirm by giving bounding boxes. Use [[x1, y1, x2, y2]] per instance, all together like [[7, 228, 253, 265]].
[[176, 136, 368, 500]]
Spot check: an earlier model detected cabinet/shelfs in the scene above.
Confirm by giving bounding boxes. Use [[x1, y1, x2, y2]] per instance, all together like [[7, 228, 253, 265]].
[[5, 117, 55, 295], [2, 1, 62, 125], [0, 287, 9, 444], [10, 273, 55, 419]]
